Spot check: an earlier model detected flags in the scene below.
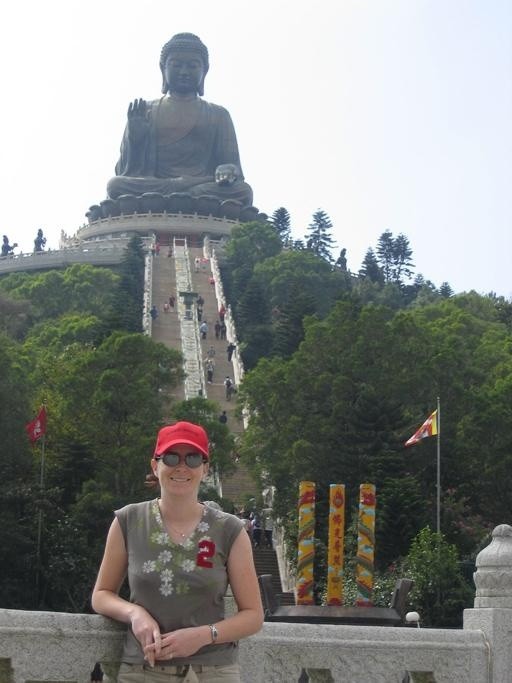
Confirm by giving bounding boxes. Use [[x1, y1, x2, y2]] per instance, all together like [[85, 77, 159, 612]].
[[403, 408, 438, 448], [24, 403, 49, 446]]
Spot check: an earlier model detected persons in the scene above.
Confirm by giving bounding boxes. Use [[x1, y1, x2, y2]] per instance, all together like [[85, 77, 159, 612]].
[[218, 303, 225, 321], [164, 302, 169, 312], [197, 304, 203, 320], [220, 320, 227, 341], [214, 320, 221, 340], [150, 304, 161, 322], [232, 432, 243, 463], [205, 344, 217, 365], [208, 275, 216, 292], [200, 319, 209, 338], [223, 375, 234, 401], [225, 342, 234, 362], [194, 254, 200, 273], [216, 409, 229, 426], [234, 494, 276, 551], [103, 28, 255, 205], [0, 234, 19, 256], [151, 241, 161, 265], [165, 245, 173, 263], [334, 246, 349, 272], [206, 359, 215, 384], [85, 419, 266, 682], [32, 227, 50, 254], [168, 294, 176, 311], [202, 256, 209, 274], [196, 295, 205, 309]]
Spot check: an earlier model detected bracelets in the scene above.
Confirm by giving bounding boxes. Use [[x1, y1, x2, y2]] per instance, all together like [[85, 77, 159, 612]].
[[209, 623, 218, 645]]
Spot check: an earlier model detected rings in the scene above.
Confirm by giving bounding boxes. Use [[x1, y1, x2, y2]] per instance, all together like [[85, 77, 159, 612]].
[[169, 654, 173, 660]]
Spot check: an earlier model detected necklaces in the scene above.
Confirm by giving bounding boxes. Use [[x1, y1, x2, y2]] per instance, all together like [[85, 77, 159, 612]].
[[164, 518, 198, 540]]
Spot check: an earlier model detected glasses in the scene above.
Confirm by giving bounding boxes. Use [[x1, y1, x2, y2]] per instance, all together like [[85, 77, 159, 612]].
[[156, 452, 206, 467]]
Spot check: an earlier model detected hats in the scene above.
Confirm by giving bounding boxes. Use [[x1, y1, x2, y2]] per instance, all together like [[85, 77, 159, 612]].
[[154, 421, 209, 457]]
